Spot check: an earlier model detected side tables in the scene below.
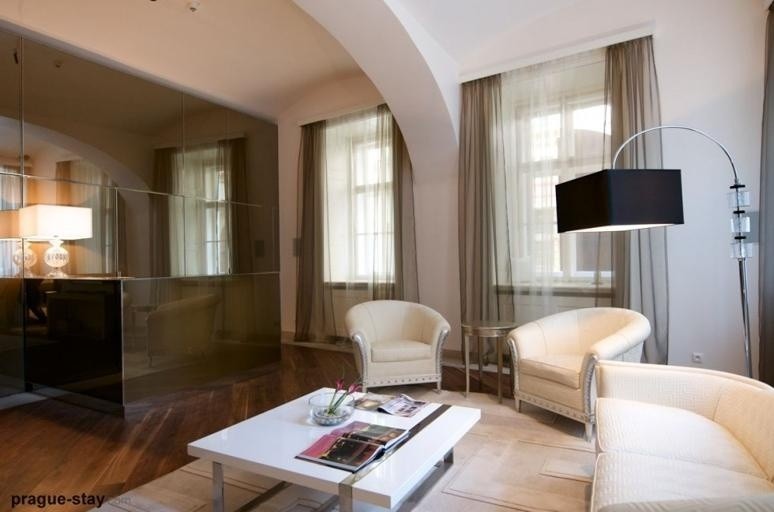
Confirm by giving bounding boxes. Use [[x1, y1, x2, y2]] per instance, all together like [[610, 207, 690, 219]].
[[130, 306, 156, 356], [461, 323, 512, 402]]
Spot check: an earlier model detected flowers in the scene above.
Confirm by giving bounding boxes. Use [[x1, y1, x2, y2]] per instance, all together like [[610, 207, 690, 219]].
[[330, 379, 362, 412]]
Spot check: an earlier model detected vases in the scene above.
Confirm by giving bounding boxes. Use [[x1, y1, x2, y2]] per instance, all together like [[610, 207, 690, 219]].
[[308, 393, 354, 425]]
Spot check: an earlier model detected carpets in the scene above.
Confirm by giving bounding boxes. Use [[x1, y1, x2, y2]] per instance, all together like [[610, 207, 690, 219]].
[[87, 389, 592, 512]]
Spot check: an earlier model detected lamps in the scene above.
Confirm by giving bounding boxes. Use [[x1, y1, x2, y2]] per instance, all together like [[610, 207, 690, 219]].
[[554, 126, 752, 378], [20, 206, 92, 277]]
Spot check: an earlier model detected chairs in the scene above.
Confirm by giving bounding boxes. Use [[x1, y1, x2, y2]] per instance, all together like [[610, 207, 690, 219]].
[[344, 299, 451, 394], [507, 308, 651, 442], [151, 293, 219, 368]]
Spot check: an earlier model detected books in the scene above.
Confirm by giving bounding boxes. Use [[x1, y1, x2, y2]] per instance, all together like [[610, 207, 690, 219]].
[[344, 391, 432, 418], [293, 420, 410, 473]]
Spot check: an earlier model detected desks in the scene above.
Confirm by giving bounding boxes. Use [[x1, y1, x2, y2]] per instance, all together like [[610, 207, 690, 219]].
[[185, 383, 482, 512]]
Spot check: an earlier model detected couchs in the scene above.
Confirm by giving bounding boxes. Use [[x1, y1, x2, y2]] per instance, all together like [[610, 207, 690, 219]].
[[589, 360, 774, 512]]
